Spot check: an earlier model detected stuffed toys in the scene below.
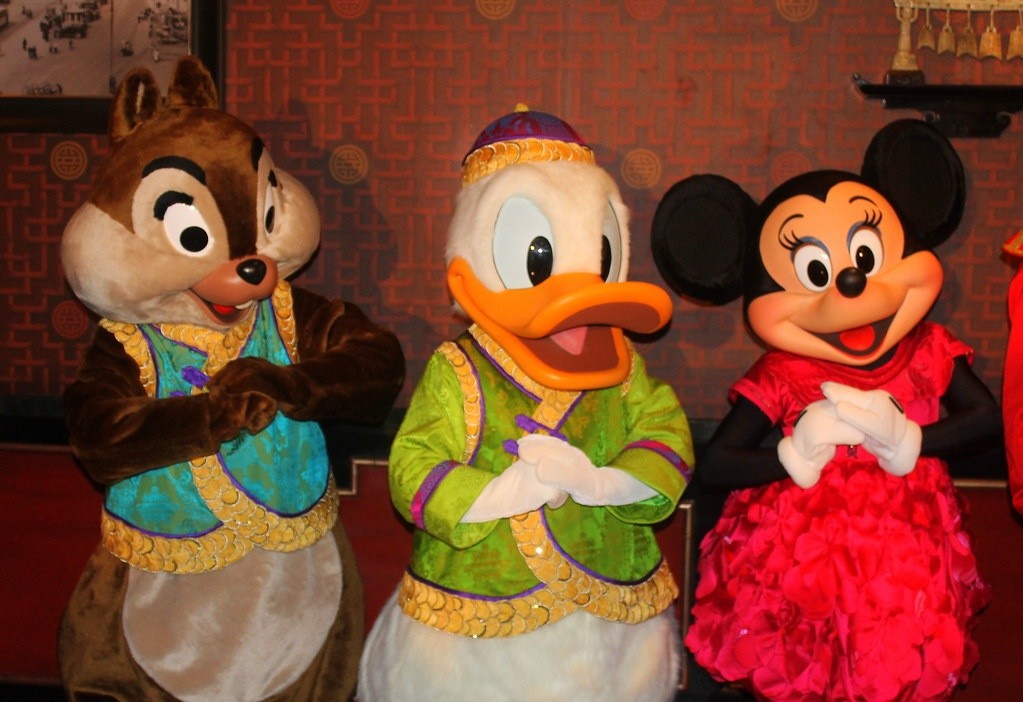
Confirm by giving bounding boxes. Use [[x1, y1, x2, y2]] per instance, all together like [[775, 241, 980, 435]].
[[356, 104, 695, 702], [58, 55, 406, 701], [652, 118, 1005, 702]]
